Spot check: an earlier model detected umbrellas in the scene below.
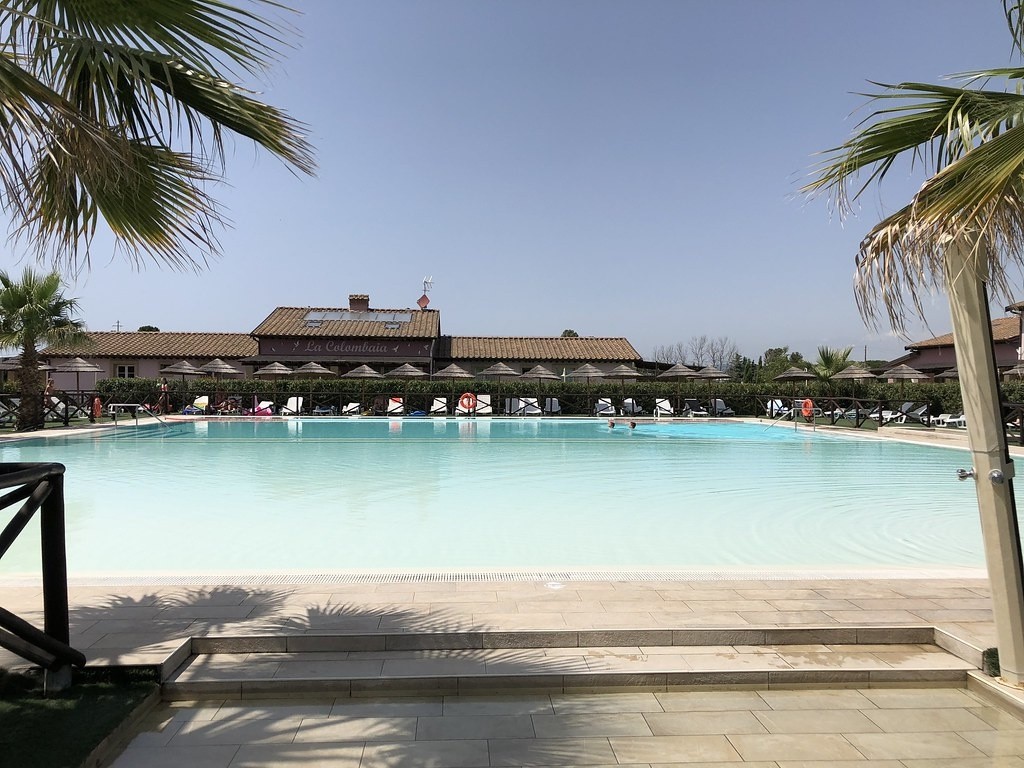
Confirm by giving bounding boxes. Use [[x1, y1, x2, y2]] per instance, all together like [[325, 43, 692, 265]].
[[934, 367, 959, 378], [828, 365, 877, 406], [50, 357, 107, 393], [341, 365, 383, 403], [519, 365, 562, 403], [194, 359, 245, 402], [877, 364, 930, 412], [656, 363, 702, 409], [602, 365, 644, 406], [253, 362, 294, 403], [566, 364, 607, 408], [0, 354, 58, 372], [432, 364, 476, 406], [773, 367, 818, 406], [292, 362, 337, 407], [687, 365, 733, 405], [384, 363, 430, 403], [1003, 364, 1024, 380], [158, 360, 205, 403], [477, 362, 521, 408]]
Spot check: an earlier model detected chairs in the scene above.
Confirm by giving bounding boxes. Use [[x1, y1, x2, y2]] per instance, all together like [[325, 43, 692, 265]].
[[596, 396, 1021, 433], [183, 396, 208, 415], [342, 402, 360, 414], [250, 401, 275, 416], [430, 397, 447, 416], [313, 406, 338, 416], [0, 396, 102, 425], [217, 396, 242, 415], [387, 398, 404, 417], [280, 397, 303, 416], [456, 394, 562, 417]]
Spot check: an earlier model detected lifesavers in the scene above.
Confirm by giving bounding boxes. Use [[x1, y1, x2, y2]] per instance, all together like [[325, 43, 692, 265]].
[[95, 397, 101, 418], [802, 399, 813, 417], [460, 393, 477, 408]]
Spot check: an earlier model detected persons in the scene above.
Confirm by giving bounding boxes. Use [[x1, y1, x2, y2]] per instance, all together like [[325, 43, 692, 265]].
[[215, 398, 238, 412], [44, 377, 59, 407], [608, 422, 615, 428], [629, 422, 636, 428], [157, 378, 169, 416]]
